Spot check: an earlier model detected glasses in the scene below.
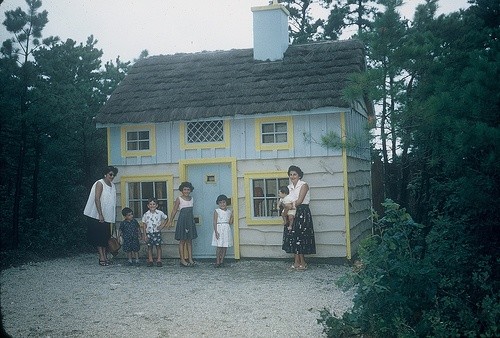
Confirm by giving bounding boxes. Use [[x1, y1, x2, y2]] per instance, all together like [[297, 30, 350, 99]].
[[107, 171, 116, 177]]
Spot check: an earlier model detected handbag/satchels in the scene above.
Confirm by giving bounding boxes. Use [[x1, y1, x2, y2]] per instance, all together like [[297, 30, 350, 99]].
[[107, 236, 120, 253]]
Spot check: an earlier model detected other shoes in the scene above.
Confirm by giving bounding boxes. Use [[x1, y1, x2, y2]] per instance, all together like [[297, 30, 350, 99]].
[[134, 262, 140, 267], [148, 262, 153, 267], [126, 261, 134, 266], [179, 260, 190, 267], [156, 262, 163, 267], [215, 263, 224, 268], [187, 261, 197, 266]]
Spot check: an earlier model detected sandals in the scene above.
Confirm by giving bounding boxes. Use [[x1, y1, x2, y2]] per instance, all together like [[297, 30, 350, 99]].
[[106, 259, 115, 265], [99, 259, 109, 267], [297, 265, 308, 272], [288, 263, 299, 271]]
